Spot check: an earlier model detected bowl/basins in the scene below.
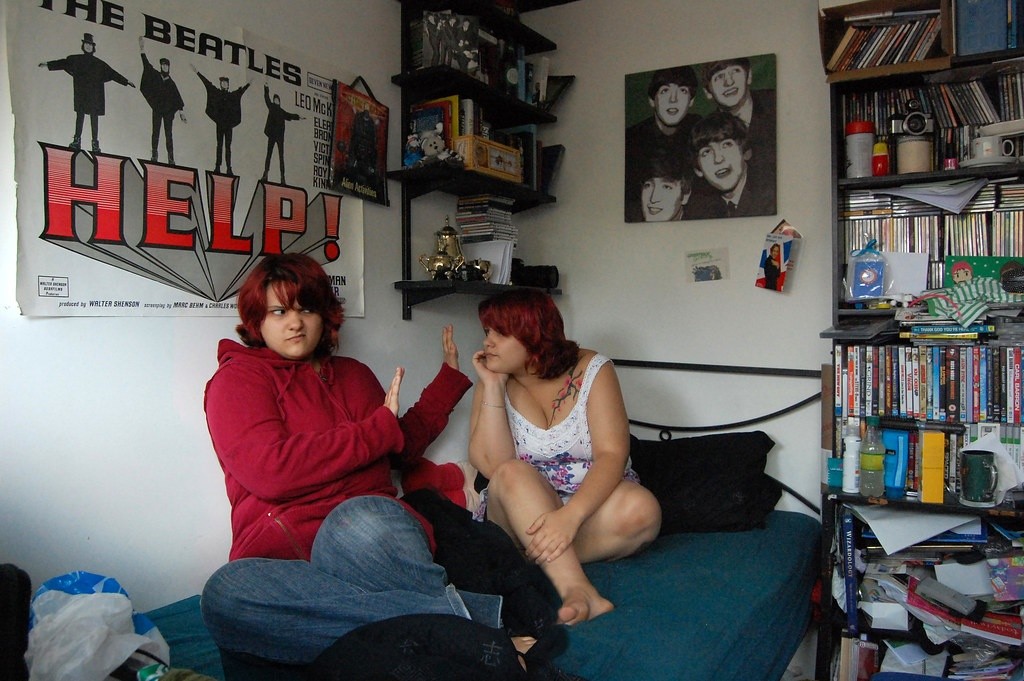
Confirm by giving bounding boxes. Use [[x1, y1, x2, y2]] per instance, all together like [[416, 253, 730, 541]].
[[976, 118, 1024, 137]]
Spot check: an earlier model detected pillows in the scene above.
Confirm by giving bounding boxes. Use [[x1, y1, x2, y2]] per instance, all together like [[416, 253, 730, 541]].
[[627, 430, 783, 535]]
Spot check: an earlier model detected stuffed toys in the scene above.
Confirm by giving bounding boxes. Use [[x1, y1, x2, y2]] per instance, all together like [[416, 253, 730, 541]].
[[404, 123, 464, 168]]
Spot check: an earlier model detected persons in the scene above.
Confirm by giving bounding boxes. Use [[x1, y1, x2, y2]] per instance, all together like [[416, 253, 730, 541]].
[[200, 253, 538, 672], [764, 243, 780, 290], [426, 14, 473, 72], [468, 289, 662, 625], [624, 57, 777, 221]]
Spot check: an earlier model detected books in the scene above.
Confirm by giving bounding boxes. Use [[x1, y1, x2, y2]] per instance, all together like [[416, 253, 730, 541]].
[[479, 29, 576, 113], [842, 73, 1024, 170], [455, 194, 519, 251], [843, 176, 1024, 288], [835, 313, 1024, 492], [409, 94, 566, 194], [409, 9, 452, 70], [826, 14, 942, 72]]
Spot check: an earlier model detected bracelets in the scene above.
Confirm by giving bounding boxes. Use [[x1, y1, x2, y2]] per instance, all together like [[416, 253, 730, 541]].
[[481, 400, 505, 408]]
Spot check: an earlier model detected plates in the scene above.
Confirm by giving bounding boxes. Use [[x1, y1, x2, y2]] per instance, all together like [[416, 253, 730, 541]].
[[959, 498, 1003, 507], [958, 157, 1016, 169]]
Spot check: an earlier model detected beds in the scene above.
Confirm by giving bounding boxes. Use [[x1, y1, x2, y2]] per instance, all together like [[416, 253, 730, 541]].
[[136, 363, 833, 680]]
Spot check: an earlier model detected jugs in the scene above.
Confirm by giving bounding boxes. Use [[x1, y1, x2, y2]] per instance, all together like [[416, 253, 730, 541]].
[[418, 215, 467, 282]]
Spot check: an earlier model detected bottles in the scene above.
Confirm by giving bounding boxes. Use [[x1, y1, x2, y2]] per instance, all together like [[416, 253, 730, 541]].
[[844, 122, 891, 179], [841, 415, 885, 498], [897, 136, 935, 174]]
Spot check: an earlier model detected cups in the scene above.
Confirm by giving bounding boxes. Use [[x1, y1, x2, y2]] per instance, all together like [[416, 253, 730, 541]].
[[960, 450, 999, 503], [970, 137, 1013, 157], [468, 257, 492, 283], [943, 158, 959, 171]]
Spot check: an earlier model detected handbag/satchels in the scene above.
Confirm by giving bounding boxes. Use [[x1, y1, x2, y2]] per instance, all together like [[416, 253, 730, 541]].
[[631, 430, 779, 533]]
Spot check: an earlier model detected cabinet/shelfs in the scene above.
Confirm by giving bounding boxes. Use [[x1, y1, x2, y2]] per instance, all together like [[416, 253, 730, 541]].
[[385, 0, 566, 323], [820, 50, 1024, 681]]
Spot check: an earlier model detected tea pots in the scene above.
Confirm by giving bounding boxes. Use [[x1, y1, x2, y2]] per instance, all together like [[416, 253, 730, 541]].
[[419, 245, 467, 282]]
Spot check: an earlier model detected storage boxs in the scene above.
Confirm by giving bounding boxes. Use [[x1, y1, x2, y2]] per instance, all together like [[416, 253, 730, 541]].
[[818, 0, 952, 85], [454, 134, 523, 184]]
